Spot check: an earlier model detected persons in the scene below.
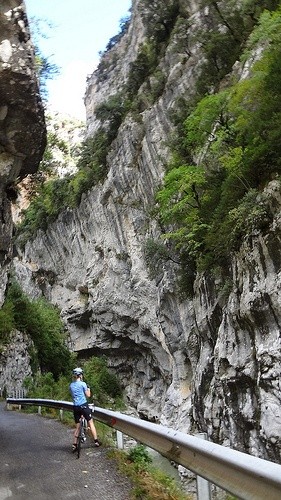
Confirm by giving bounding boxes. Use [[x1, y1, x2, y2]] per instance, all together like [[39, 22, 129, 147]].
[[69, 367, 102, 451]]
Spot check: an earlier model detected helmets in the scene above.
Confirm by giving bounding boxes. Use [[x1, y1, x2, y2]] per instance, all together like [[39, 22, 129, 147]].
[[73, 368, 83, 376]]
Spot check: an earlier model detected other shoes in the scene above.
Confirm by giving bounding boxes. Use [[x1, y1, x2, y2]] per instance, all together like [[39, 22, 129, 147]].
[[72, 445, 76, 452], [95, 441, 101, 446]]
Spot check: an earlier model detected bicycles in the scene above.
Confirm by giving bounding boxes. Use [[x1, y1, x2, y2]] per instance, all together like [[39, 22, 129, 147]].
[[74, 400, 94, 458]]
